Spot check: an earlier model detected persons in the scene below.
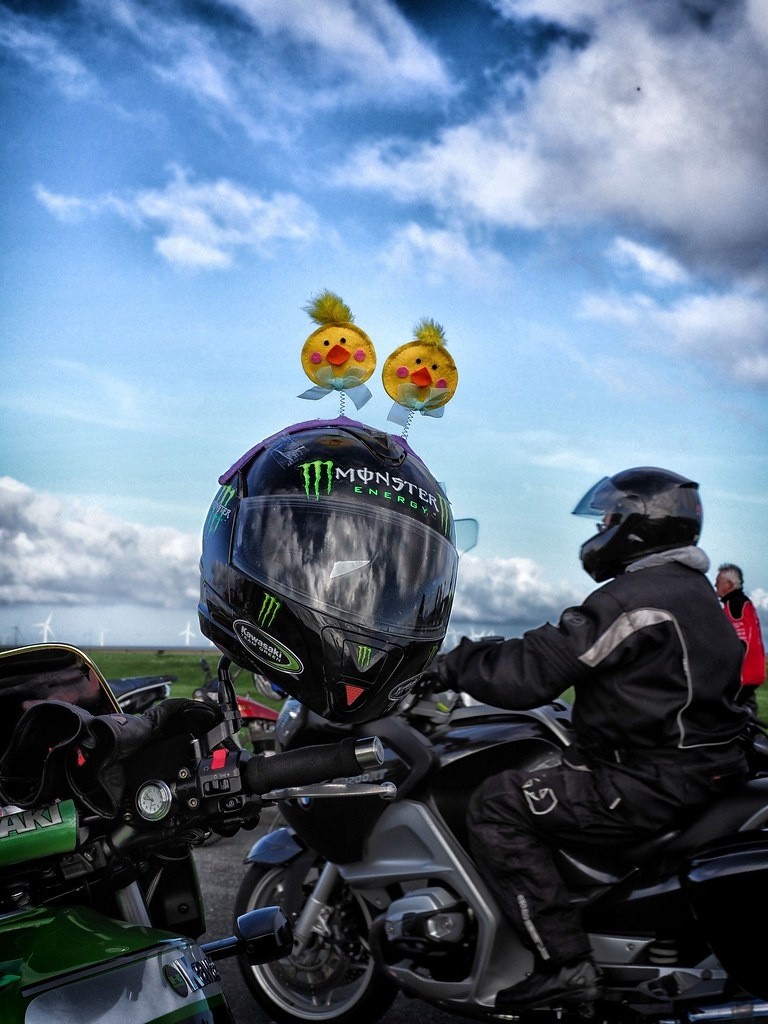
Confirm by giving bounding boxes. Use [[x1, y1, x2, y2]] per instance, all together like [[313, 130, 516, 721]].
[[432, 466, 752, 1006], [713, 563, 767, 712]]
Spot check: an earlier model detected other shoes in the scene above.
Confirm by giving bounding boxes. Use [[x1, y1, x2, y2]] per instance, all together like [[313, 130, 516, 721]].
[[495, 962, 612, 1011]]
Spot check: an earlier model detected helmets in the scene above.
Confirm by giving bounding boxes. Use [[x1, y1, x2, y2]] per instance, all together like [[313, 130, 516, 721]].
[[197, 415, 460, 725], [570, 466, 703, 583]]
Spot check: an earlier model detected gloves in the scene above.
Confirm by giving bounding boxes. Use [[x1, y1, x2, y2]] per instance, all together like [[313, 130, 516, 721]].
[[0, 702, 91, 807], [410, 653, 450, 700], [64, 697, 217, 824]]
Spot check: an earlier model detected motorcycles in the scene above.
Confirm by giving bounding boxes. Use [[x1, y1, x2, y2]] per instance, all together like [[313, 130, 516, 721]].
[[0, 517, 768, 1022]]
